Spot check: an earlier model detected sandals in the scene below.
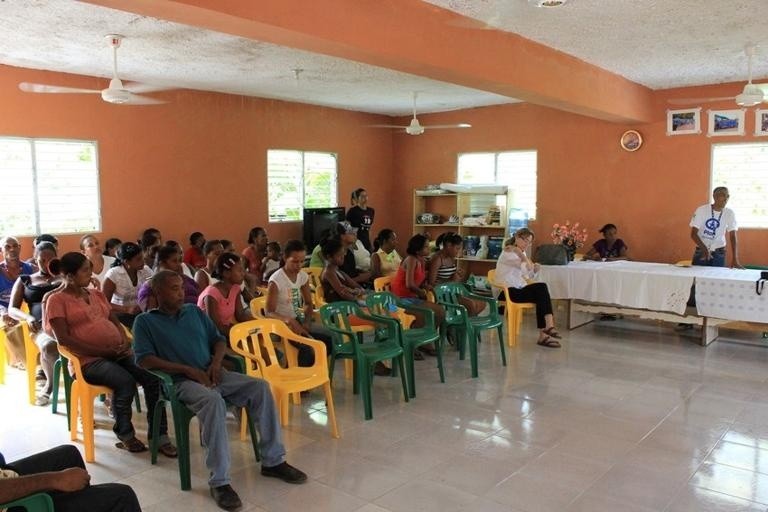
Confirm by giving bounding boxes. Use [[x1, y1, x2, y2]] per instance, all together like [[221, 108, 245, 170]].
[[11, 362, 25, 370], [542, 326, 562, 340], [103, 398, 115, 419], [34, 391, 50, 406], [79, 414, 96, 430], [537, 336, 561, 348]]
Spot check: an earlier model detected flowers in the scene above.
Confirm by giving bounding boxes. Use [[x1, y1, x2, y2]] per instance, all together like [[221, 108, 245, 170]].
[[550, 220, 588, 249]]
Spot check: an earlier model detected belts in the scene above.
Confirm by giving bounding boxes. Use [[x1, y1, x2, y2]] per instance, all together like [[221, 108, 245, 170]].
[[695, 246, 725, 252]]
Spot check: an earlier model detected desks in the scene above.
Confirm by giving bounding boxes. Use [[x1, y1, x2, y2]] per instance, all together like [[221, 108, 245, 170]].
[[539, 261, 768, 346]]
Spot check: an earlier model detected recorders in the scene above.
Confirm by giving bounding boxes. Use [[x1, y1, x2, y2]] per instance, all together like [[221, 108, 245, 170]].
[[417, 213, 440, 224]]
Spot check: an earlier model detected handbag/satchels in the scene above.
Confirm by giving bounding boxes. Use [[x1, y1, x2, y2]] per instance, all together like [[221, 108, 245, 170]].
[[760, 270, 768, 280], [533, 243, 569, 265]]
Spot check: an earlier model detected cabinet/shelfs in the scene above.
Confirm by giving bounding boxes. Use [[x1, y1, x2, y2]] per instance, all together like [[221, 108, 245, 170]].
[[412, 187, 508, 283]]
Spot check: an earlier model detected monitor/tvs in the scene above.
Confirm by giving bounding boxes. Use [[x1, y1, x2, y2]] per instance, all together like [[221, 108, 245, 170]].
[[303, 207, 346, 254]]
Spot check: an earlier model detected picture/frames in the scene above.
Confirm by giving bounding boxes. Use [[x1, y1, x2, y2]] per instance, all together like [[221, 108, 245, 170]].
[[754, 108, 768, 136], [708, 109, 745, 136], [667, 108, 700, 135]]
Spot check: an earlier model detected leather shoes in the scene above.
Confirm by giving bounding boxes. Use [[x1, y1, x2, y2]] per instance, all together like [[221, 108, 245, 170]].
[[260, 461, 308, 484], [210, 482, 243, 512]]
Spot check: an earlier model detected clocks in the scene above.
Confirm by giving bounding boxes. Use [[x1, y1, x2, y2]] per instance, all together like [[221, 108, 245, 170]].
[[621, 130, 642, 152]]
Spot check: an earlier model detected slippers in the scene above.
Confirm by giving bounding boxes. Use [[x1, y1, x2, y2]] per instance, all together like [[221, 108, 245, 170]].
[[115, 437, 149, 453], [158, 443, 177, 458]]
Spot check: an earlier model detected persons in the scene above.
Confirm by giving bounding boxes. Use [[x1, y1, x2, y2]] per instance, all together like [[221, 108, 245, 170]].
[[129, 269, 308, 510], [581, 223, 629, 322], [672, 187, 744, 333], [1, 222, 486, 457], [495, 227, 564, 349], [344, 186, 375, 249], [0, 444, 140, 510]]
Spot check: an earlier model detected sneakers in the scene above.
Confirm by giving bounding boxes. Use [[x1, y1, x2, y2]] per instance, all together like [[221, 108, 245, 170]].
[[36, 369, 47, 381], [374, 361, 392, 376], [413, 350, 425, 361], [416, 345, 436, 356]]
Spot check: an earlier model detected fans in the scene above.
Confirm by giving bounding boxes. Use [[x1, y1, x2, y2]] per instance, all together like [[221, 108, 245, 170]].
[[18, 35, 184, 106], [360, 90, 472, 136], [667, 46, 768, 106]]
